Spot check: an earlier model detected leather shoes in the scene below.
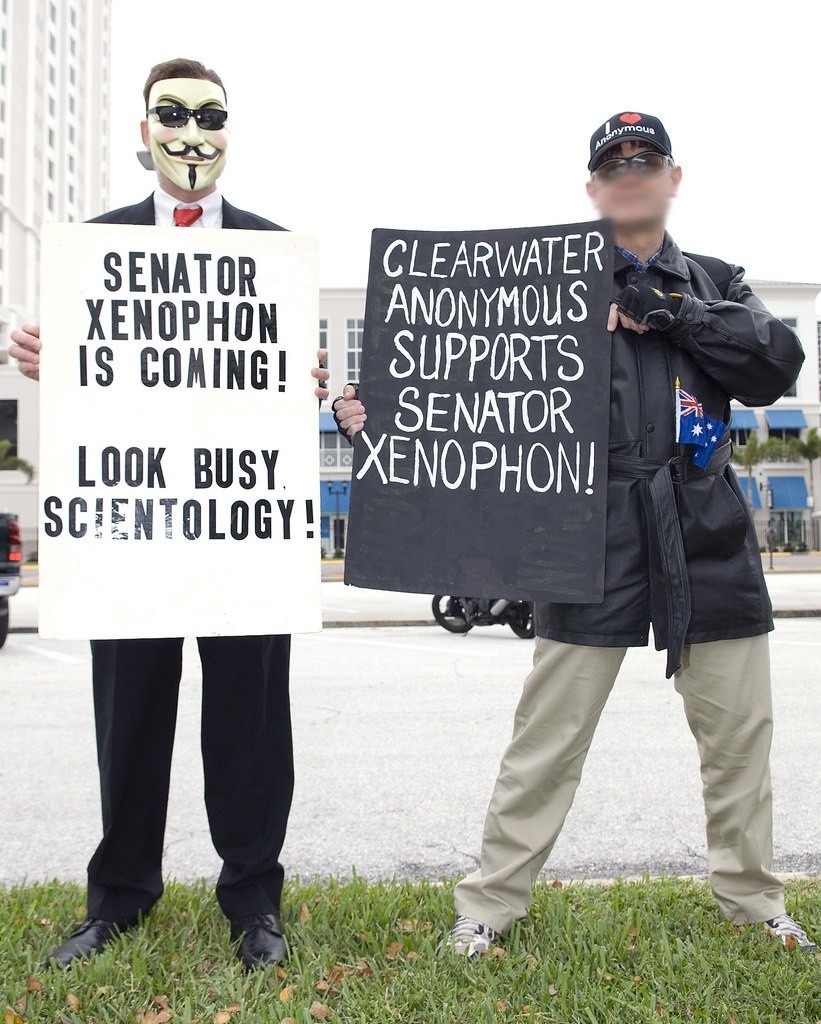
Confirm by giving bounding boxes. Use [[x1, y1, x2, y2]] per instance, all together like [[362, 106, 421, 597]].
[[46, 917, 127, 970], [229, 914, 288, 970]]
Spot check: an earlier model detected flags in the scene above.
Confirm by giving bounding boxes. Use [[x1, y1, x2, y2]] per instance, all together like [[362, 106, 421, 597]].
[[676, 389, 725, 470]]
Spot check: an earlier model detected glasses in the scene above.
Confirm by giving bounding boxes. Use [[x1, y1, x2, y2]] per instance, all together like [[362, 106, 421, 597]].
[[145, 105, 228, 131], [591, 151, 673, 184]]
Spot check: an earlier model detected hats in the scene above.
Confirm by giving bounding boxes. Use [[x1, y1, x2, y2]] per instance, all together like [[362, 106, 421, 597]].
[[587, 112, 672, 171]]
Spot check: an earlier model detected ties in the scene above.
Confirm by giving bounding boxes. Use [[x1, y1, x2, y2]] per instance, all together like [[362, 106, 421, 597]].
[[174, 207, 203, 227]]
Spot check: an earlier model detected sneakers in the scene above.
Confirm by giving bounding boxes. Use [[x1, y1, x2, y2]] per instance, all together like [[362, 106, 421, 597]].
[[737, 915, 813, 953], [434, 915, 498, 964]]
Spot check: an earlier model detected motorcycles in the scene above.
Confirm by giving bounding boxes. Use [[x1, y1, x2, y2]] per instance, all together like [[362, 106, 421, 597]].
[[432, 595, 536, 639]]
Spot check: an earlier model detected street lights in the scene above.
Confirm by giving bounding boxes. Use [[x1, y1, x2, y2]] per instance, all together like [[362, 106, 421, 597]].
[[326, 480, 348, 558]]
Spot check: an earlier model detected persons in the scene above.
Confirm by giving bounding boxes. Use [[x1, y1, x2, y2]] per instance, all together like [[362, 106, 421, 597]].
[[9, 59, 328, 976], [329, 112, 817, 961]]
